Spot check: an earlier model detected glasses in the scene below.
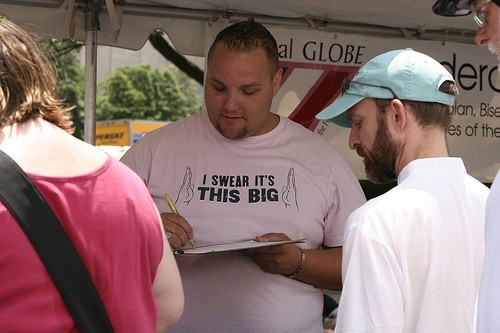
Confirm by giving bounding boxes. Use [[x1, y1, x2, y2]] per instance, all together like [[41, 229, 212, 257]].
[[471, 1, 491, 28], [341, 78, 398, 99]]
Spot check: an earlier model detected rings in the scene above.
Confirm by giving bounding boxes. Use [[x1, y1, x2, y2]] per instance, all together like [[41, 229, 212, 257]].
[[166, 229, 172, 239]]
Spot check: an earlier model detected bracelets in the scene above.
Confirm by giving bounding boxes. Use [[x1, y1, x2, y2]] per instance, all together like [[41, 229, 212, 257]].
[[287, 247, 306, 279]]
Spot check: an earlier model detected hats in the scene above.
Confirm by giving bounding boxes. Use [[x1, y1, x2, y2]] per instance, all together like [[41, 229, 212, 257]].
[[432, 0, 472, 17], [314, 48, 457, 128]]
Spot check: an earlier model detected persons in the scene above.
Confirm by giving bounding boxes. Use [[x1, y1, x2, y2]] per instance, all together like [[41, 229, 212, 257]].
[[0, 13, 188, 333], [430, 0, 500, 333], [117, 21, 370, 333], [313, 47, 490, 333]]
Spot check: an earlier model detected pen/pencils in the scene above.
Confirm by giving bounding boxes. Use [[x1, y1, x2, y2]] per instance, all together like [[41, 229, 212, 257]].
[[163, 193, 197, 249]]
